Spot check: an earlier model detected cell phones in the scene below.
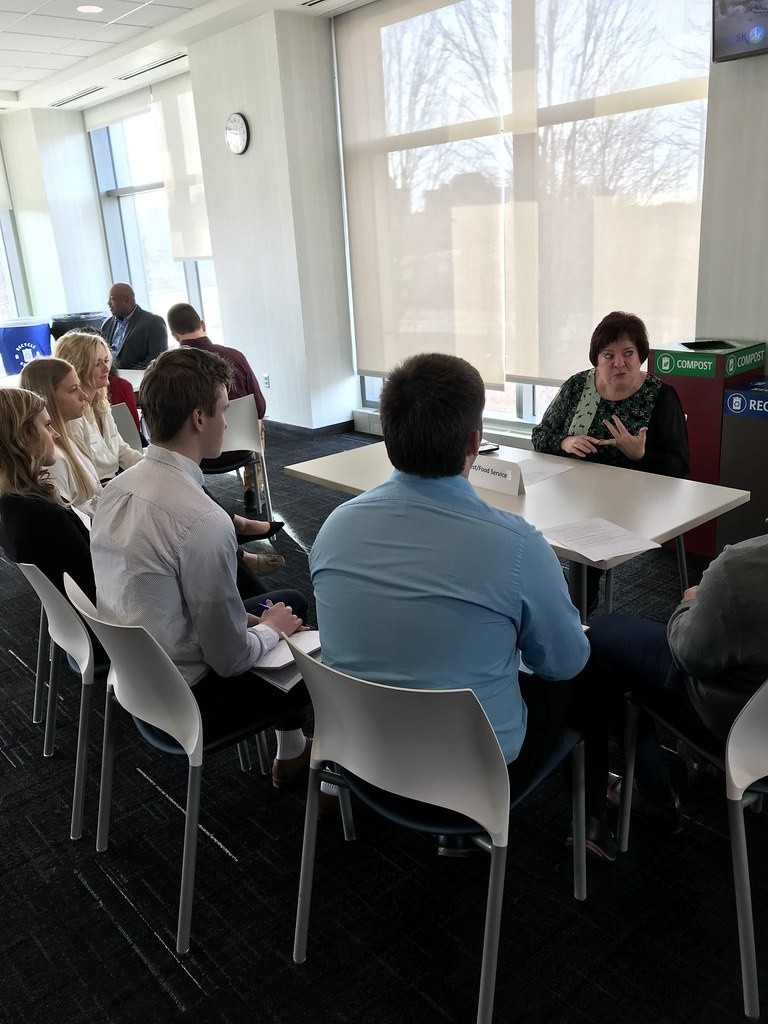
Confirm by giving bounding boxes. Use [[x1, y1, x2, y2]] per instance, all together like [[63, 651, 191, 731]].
[[478, 445, 499, 454]]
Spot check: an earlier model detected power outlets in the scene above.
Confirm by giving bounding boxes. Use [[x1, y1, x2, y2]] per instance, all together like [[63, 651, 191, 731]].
[[264, 375, 269, 388]]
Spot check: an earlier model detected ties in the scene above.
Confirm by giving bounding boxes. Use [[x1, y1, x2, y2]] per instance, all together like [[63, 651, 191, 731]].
[[111, 319, 130, 352]]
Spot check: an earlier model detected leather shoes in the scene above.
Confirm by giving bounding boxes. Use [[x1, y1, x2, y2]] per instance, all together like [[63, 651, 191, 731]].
[[235, 521, 284, 545], [565, 814, 620, 864], [437, 832, 514, 863], [318, 791, 357, 826], [603, 770, 686, 823], [272, 736, 314, 789]]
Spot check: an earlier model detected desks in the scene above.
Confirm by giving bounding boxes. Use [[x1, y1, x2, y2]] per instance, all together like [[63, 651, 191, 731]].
[[284, 437, 751, 626], [1, 369, 146, 406]]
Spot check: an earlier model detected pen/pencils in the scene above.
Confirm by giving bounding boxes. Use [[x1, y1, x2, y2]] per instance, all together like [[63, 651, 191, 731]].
[[258, 602, 271, 609]]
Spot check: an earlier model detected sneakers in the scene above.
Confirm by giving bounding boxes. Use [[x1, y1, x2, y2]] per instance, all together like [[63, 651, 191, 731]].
[[243, 487, 266, 512], [251, 553, 285, 577]]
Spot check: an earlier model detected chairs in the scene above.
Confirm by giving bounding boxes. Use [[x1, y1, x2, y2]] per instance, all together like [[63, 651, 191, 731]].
[[0, 369, 768, 1024]]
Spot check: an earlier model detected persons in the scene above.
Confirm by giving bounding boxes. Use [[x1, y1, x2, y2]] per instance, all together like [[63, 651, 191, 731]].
[[0, 329, 285, 666], [167, 304, 268, 514], [98, 282, 166, 370], [305, 355, 619, 878], [89, 351, 313, 792], [574, 534, 768, 820], [529, 310, 690, 622]]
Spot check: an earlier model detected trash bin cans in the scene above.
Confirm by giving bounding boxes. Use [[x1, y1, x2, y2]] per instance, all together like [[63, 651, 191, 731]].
[[0, 312, 107, 376], [648, 337, 768, 554]]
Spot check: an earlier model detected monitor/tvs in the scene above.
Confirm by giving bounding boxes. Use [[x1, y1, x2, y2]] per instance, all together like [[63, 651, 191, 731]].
[[712, 0, 768, 63]]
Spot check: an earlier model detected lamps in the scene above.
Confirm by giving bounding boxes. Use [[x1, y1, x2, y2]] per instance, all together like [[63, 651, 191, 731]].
[[224, 113, 249, 155]]
